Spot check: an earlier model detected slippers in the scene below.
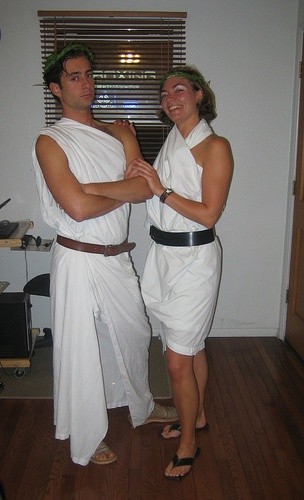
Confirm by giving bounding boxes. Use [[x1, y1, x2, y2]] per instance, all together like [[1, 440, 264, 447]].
[[161, 422, 209, 438], [165, 446, 201, 479]]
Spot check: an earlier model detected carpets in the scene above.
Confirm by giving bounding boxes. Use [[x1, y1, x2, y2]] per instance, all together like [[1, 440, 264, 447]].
[[0, 336, 174, 399]]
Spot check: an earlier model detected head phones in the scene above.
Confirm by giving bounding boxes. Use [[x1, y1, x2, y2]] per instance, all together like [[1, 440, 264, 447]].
[[20, 235, 41, 249]]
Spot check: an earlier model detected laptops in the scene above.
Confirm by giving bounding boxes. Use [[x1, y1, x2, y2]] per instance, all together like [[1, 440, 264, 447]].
[[0, 198, 19, 239]]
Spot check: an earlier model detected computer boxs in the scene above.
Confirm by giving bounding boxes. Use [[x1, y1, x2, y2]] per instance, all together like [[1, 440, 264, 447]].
[[0, 292, 33, 359]]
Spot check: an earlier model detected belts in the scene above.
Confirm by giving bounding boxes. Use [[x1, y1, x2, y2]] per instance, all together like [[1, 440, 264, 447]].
[[56, 235, 136, 257], [149, 225, 215, 247]]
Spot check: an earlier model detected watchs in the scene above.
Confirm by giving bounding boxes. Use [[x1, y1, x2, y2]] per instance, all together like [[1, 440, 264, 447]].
[[159, 188, 173, 204]]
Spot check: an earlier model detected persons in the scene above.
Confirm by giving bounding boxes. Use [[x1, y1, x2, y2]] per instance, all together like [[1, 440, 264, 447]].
[[123, 66, 236, 483], [32, 44, 181, 467]]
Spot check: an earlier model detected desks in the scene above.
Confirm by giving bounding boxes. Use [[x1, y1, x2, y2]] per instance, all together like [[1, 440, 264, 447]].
[[0, 220, 40, 378]]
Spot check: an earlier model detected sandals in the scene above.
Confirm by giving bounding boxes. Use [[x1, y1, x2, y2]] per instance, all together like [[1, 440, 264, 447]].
[[89, 441, 118, 464], [130, 403, 179, 424]]
